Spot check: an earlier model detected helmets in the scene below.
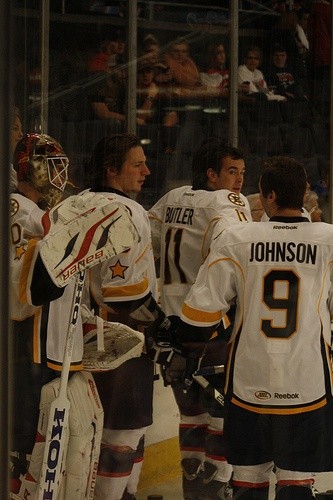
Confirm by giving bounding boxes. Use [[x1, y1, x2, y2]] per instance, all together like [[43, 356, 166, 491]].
[[12, 132, 66, 180]]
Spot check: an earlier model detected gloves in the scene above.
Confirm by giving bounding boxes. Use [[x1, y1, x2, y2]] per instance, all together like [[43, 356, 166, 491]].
[[150, 316, 176, 362], [165, 338, 207, 385]]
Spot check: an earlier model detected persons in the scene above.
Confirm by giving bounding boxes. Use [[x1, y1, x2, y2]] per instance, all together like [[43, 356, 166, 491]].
[[7, 0, 333, 500], [170, 156, 333, 500]]
